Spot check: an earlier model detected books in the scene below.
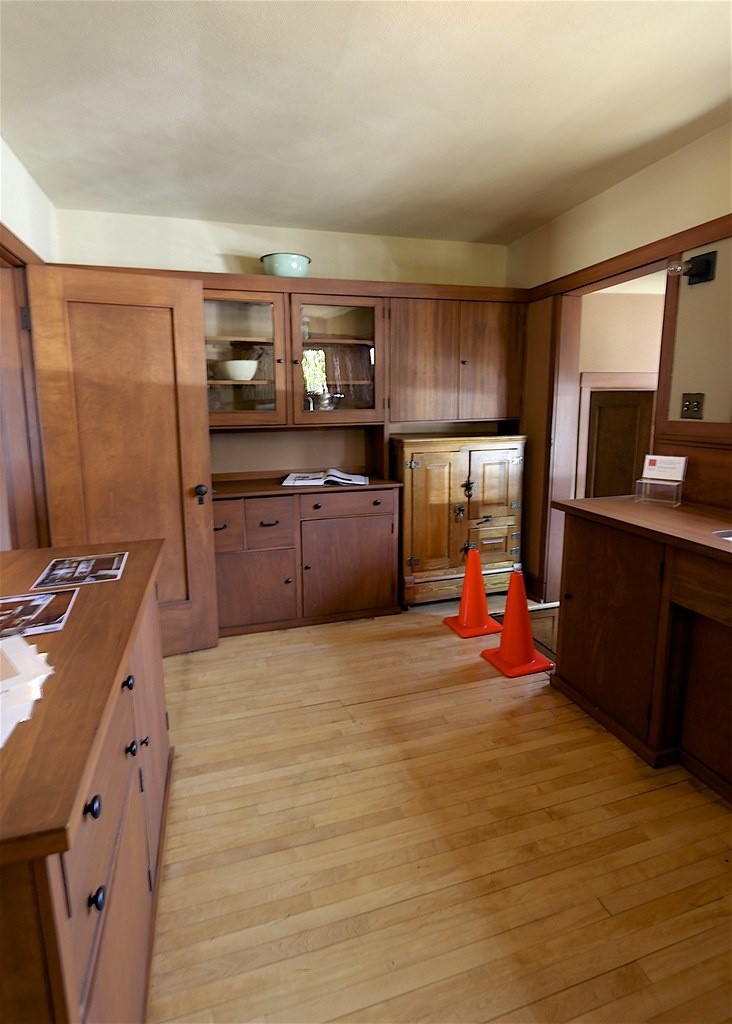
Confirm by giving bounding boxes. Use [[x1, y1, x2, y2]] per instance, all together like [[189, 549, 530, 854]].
[[281, 468, 370, 487]]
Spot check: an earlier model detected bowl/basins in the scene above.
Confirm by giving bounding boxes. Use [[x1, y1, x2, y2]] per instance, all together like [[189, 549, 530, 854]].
[[208, 360, 262, 381], [259, 252, 311, 277]]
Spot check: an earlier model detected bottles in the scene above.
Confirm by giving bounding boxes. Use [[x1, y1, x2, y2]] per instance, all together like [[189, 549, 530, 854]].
[[302, 316, 311, 339]]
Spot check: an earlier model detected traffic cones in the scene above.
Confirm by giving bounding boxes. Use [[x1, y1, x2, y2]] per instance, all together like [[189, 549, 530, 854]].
[[443, 548, 503, 638], [479, 572, 556, 679]]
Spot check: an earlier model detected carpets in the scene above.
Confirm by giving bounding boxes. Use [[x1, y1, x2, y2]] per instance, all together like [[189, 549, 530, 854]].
[[490, 606, 559, 661]]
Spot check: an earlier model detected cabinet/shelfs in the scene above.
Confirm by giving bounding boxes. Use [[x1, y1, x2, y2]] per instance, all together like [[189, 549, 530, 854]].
[[0, 534, 175, 1024], [389, 298, 523, 423], [197, 287, 386, 426], [392, 432, 528, 607], [550, 495, 732, 808], [208, 473, 403, 640]]
